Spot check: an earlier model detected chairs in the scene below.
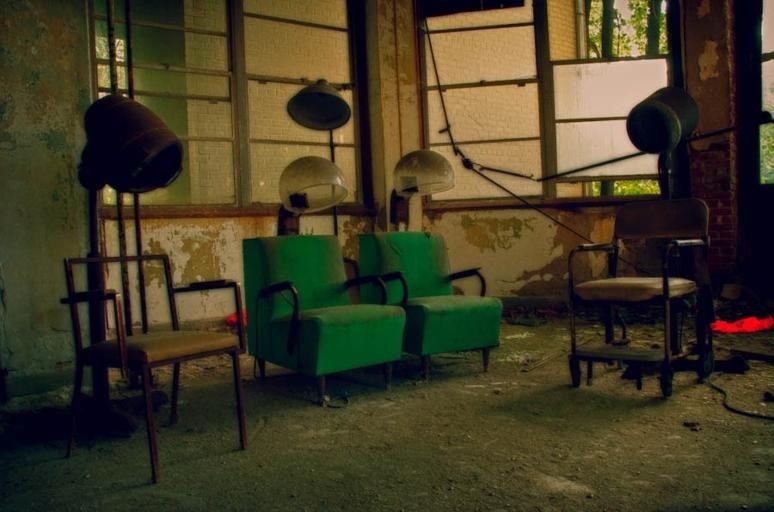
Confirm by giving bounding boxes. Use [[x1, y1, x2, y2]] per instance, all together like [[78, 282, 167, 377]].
[[59, 252, 249, 482], [568, 196, 715, 397]]
